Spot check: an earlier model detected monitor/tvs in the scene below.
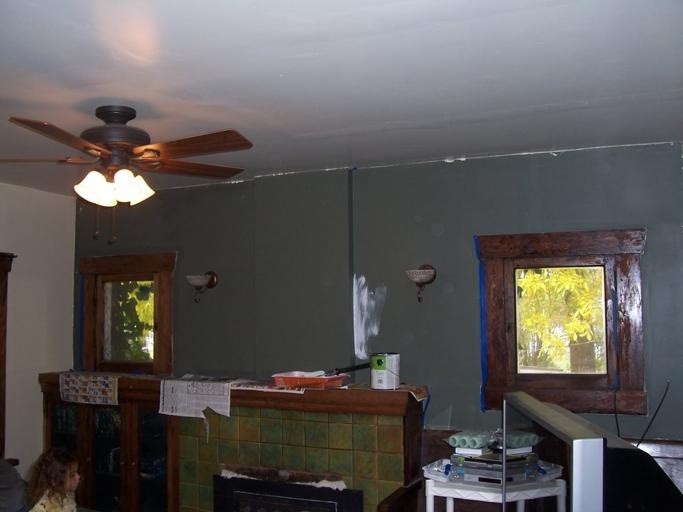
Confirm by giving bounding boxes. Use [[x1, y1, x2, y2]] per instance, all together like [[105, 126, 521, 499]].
[[501, 391, 683, 511]]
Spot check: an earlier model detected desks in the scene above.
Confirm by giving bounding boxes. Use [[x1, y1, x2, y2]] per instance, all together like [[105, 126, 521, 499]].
[[425, 479, 504, 512]]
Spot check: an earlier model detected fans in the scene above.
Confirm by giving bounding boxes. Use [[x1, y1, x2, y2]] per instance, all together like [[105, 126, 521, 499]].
[[0, 107, 254, 181]]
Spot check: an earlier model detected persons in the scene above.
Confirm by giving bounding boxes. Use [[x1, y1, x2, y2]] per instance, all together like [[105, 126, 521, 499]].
[[25, 447, 80, 511]]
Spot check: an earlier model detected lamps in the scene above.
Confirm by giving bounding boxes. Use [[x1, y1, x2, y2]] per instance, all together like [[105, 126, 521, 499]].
[[74, 169, 156, 208], [186, 271, 216, 303], [405, 264, 437, 302]]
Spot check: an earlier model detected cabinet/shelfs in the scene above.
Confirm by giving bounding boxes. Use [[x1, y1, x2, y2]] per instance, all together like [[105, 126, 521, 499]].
[[39, 371, 173, 512]]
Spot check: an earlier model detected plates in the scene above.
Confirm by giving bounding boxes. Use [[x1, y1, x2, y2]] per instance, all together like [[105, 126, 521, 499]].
[[274, 371, 348, 389], [422, 459, 453, 483]]
